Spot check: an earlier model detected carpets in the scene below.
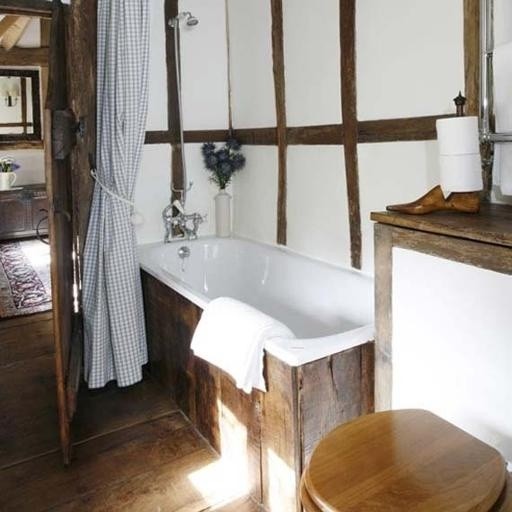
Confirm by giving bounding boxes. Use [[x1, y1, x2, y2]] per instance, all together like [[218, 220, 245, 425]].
[[0, 239, 53, 319]]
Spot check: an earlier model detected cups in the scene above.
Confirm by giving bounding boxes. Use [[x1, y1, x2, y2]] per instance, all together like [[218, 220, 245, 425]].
[[0, 172, 16, 188]]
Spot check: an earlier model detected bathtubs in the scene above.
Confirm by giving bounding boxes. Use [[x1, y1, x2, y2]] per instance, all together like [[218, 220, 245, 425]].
[[136, 235, 375, 369]]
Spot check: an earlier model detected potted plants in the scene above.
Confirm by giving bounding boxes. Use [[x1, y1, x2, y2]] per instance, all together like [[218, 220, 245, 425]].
[[202, 137, 247, 240]]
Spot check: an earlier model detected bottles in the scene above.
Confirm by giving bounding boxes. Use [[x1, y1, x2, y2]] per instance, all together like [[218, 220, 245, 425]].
[[214, 189, 232, 237]]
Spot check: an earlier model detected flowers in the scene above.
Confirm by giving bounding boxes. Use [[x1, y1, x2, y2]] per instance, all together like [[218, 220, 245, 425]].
[[1, 156, 20, 171]]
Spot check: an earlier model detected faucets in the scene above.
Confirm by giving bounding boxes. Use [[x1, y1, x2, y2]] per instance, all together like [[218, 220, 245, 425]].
[[179, 222, 191, 240]]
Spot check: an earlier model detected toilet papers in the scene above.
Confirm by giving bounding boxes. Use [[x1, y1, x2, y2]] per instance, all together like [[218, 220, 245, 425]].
[[436, 116, 486, 195]]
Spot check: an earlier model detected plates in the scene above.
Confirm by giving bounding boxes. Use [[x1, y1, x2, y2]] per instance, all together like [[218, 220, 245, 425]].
[[1, 187, 25, 191]]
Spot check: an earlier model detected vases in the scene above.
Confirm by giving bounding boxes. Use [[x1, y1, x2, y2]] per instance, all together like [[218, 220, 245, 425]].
[[1, 172, 17, 190]]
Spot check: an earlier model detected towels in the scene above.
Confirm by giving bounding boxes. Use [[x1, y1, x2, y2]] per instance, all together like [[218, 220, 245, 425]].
[[190, 296, 298, 394]]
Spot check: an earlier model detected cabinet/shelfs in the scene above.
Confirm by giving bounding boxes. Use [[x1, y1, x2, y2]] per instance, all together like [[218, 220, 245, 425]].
[[0, 183, 51, 240]]
